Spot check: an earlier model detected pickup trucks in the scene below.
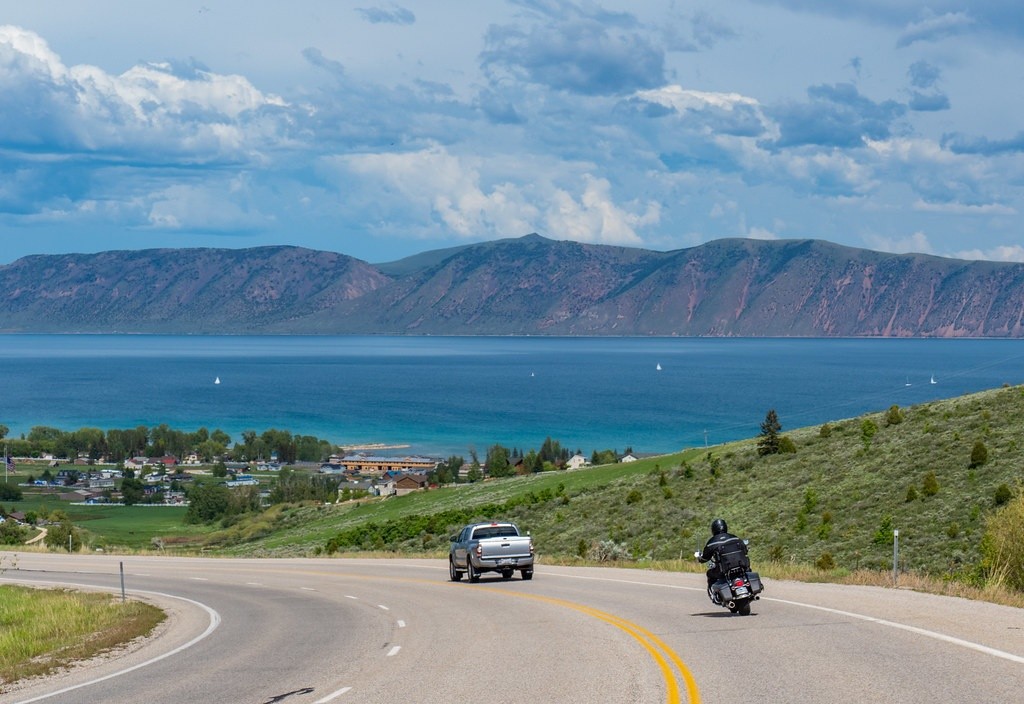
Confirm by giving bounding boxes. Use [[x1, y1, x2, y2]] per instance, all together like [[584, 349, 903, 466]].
[[448, 523, 534, 584]]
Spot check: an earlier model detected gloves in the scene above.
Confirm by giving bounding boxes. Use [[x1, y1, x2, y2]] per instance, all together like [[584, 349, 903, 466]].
[[698, 556, 708, 563]]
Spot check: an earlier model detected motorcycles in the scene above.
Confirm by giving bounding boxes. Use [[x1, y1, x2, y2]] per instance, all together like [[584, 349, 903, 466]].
[[694, 540, 764, 616]]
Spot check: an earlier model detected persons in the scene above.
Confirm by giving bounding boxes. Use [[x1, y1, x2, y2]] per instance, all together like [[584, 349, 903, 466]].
[[698, 518, 752, 589]]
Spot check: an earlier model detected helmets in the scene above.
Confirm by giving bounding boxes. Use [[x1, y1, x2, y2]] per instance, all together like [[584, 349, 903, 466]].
[[711, 519, 728, 536]]
